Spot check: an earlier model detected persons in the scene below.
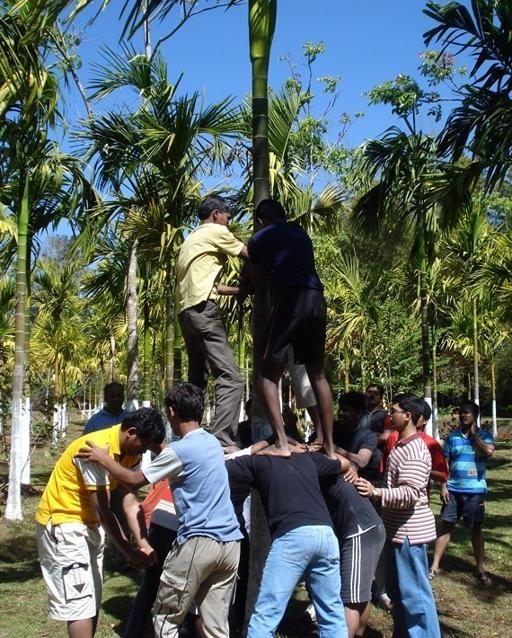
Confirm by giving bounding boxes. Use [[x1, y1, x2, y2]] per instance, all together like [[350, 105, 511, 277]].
[[81, 381, 131, 437], [225, 399, 386, 638], [232, 198, 339, 463], [356, 393, 441, 638], [74, 381, 244, 637], [173, 190, 252, 454], [424, 398, 496, 591], [336, 384, 449, 611], [37, 408, 165, 637]]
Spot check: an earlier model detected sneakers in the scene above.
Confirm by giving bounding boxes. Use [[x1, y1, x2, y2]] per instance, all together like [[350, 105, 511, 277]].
[[111, 569, 146, 577]]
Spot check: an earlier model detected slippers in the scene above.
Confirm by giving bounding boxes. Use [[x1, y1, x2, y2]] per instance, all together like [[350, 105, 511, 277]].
[[429, 568, 440, 580], [475, 569, 492, 586]]
